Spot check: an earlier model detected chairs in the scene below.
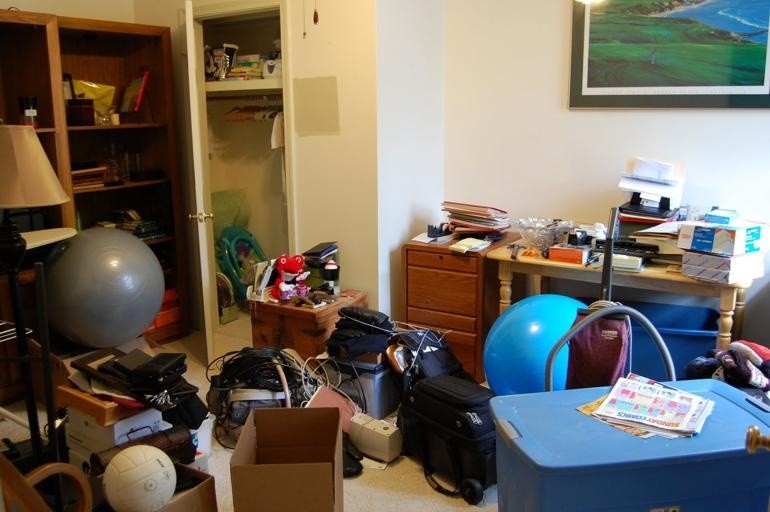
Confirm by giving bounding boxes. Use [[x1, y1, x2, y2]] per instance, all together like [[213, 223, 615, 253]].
[[0, 452, 93, 512]]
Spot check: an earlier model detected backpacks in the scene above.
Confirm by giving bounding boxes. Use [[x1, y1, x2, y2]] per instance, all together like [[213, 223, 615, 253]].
[[385, 328, 480, 393]]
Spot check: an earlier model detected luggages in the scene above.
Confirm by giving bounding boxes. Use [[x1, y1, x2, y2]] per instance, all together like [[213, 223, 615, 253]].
[[396, 375, 498, 505]]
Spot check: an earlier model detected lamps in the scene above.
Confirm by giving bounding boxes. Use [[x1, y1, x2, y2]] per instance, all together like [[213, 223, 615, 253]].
[[0, 124, 73, 468]]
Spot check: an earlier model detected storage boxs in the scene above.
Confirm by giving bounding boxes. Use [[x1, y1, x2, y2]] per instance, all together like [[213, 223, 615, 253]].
[[94, 457, 219, 512], [229, 405, 344, 512], [576, 296, 721, 383], [488, 373, 770, 512]]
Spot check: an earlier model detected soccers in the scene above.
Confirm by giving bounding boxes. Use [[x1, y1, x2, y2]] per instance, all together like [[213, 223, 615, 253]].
[[102, 445, 177, 512]]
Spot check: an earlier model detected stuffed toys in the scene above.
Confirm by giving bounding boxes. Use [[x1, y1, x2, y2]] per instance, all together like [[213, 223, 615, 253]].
[[271, 255, 310, 300]]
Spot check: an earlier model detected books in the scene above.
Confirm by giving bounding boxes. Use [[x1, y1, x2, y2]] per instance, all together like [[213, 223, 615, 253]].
[[72, 143, 142, 190], [302, 240, 340, 260], [441, 200, 511, 241], [304, 385, 362, 435], [618, 192, 671, 224], [98, 205, 145, 230], [631, 230, 685, 266]]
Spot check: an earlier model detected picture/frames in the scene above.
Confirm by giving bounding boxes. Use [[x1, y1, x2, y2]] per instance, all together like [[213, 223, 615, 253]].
[[566, 0, 770, 112]]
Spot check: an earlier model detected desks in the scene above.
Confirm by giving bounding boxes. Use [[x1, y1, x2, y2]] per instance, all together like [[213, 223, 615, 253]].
[[486, 236, 749, 359]]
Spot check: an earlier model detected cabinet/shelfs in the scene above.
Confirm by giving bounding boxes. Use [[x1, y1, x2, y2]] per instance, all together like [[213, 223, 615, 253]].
[[0, 7, 189, 405], [242, 286, 367, 368], [399, 238, 501, 384], [190, 1, 294, 370]]
